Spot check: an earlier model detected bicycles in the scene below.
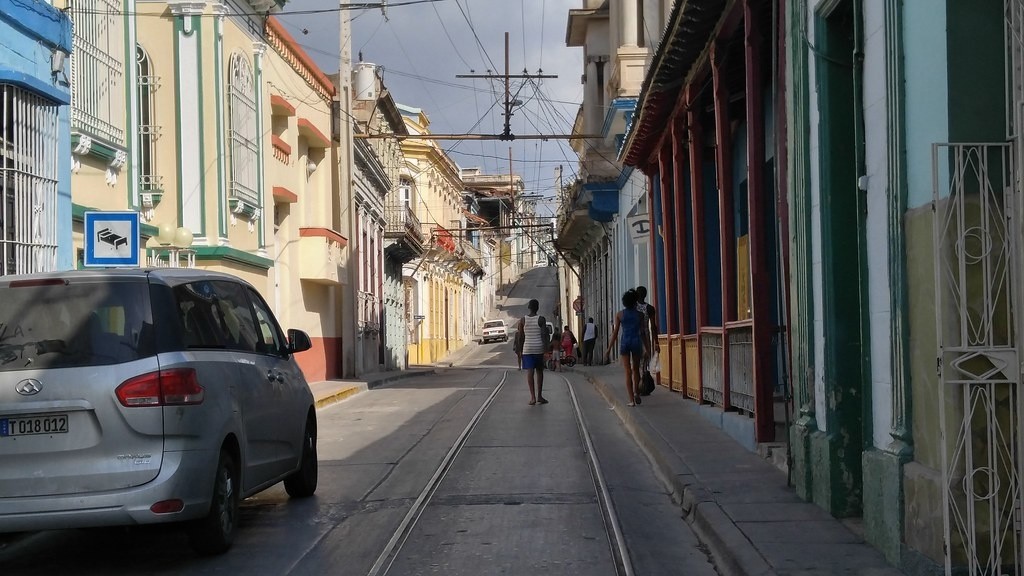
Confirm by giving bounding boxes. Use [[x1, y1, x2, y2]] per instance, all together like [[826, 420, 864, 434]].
[[547, 349, 576, 371]]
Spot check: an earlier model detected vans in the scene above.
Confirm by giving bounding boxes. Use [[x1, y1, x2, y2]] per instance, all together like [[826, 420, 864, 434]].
[[1, 266, 322, 554]]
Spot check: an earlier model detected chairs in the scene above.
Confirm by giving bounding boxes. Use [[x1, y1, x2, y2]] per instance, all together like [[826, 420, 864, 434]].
[[46, 310, 242, 358]]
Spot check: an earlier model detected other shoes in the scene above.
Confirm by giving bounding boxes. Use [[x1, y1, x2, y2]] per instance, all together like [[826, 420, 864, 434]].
[[528, 397, 549, 404], [626, 395, 641, 406], [583, 363, 592, 367]]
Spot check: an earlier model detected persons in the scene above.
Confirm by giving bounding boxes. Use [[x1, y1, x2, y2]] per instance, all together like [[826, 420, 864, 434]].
[[580, 318, 598, 366], [605, 288, 651, 407], [631, 285, 661, 395], [517, 298, 549, 406], [562, 325, 573, 358], [513, 321, 563, 373]]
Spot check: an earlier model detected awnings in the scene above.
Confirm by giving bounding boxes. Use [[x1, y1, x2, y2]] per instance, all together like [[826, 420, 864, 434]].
[[464, 211, 491, 230]]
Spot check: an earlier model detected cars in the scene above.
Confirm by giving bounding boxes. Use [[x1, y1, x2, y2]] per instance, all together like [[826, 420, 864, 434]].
[[481, 319, 509, 342]]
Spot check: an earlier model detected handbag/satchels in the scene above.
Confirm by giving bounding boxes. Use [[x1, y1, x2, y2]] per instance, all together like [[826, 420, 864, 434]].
[[648, 352, 662, 374], [570, 331, 576, 344], [638, 361, 655, 396]]
